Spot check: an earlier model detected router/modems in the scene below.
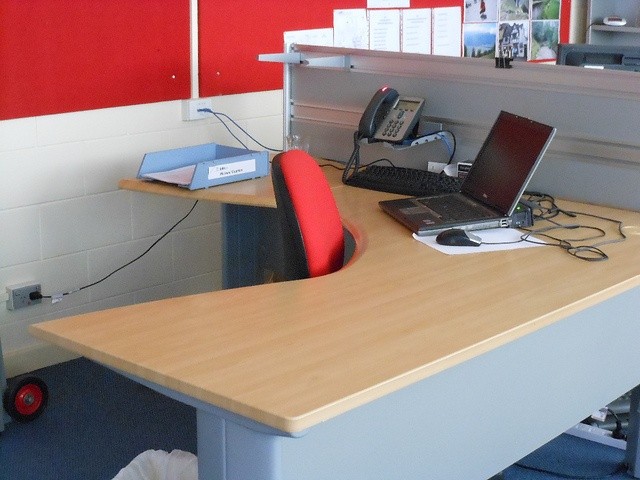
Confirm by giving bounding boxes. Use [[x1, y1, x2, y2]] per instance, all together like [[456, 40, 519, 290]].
[[603, 17, 626, 26]]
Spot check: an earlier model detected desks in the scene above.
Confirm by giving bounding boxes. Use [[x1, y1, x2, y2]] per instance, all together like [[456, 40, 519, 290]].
[[27, 152, 639, 435]]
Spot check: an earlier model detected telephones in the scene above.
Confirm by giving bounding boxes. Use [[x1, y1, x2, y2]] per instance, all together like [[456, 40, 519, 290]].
[[342, 87, 425, 184]]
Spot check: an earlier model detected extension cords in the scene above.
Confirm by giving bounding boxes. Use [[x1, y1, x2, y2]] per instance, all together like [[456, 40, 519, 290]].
[[564, 422, 627, 452]]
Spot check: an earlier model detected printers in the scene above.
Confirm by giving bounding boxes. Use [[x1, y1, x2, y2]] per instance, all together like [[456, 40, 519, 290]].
[[556, 43, 640, 74]]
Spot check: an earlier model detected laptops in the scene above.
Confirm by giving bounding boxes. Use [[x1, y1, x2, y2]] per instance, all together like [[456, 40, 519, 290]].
[[378, 110, 556, 236]]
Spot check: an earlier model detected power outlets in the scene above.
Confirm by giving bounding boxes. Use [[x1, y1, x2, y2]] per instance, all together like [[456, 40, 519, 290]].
[[6, 281, 42, 310]]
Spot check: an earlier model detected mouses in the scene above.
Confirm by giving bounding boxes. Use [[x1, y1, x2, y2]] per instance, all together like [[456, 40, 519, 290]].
[[436, 229, 482, 248]]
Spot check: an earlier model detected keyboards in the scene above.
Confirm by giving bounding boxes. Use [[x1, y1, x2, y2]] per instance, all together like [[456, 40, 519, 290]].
[[346, 163, 468, 198]]
